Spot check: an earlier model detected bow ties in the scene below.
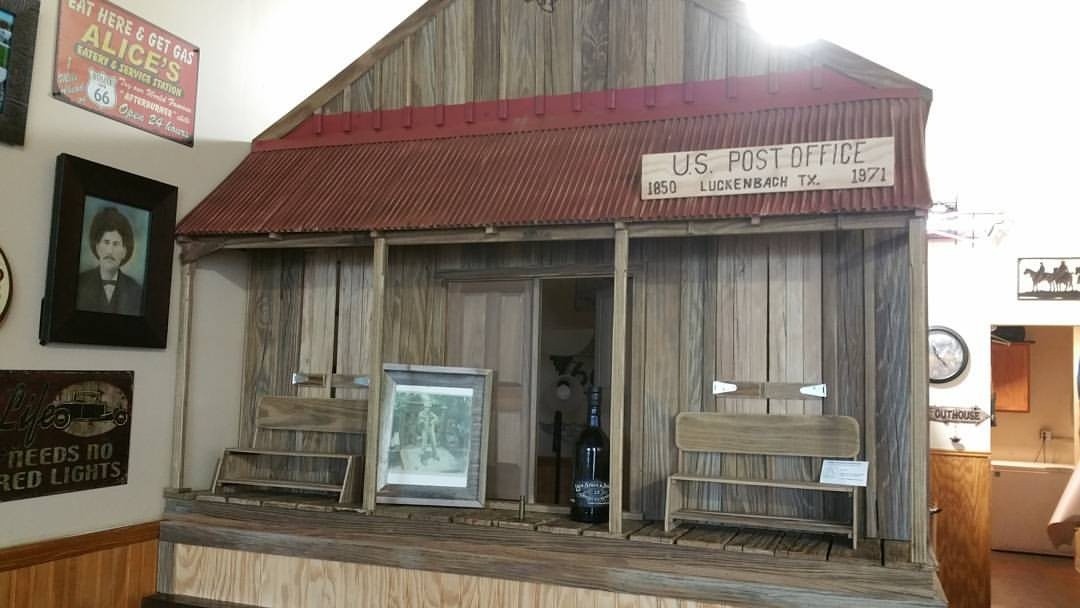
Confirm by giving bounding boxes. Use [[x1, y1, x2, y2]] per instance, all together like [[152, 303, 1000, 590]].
[[102, 280, 119, 286]]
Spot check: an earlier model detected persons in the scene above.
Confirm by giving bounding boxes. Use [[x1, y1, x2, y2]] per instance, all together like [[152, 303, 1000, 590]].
[[75, 206, 142, 317], [417, 401, 439, 462]]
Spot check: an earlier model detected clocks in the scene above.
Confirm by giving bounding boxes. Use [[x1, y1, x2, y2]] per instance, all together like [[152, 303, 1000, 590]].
[[927, 325, 969, 383]]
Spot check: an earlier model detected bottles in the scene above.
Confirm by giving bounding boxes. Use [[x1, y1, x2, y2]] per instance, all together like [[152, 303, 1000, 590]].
[[569, 385, 609, 523]]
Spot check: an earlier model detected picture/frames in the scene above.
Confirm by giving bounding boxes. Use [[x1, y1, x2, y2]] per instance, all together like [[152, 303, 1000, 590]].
[[0, 0, 42, 144], [377, 364, 494, 507], [38, 154, 180, 352]]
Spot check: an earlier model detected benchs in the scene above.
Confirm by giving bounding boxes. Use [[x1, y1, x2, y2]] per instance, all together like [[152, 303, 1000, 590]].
[[665, 410, 866, 547], [209, 394, 367, 506]]
